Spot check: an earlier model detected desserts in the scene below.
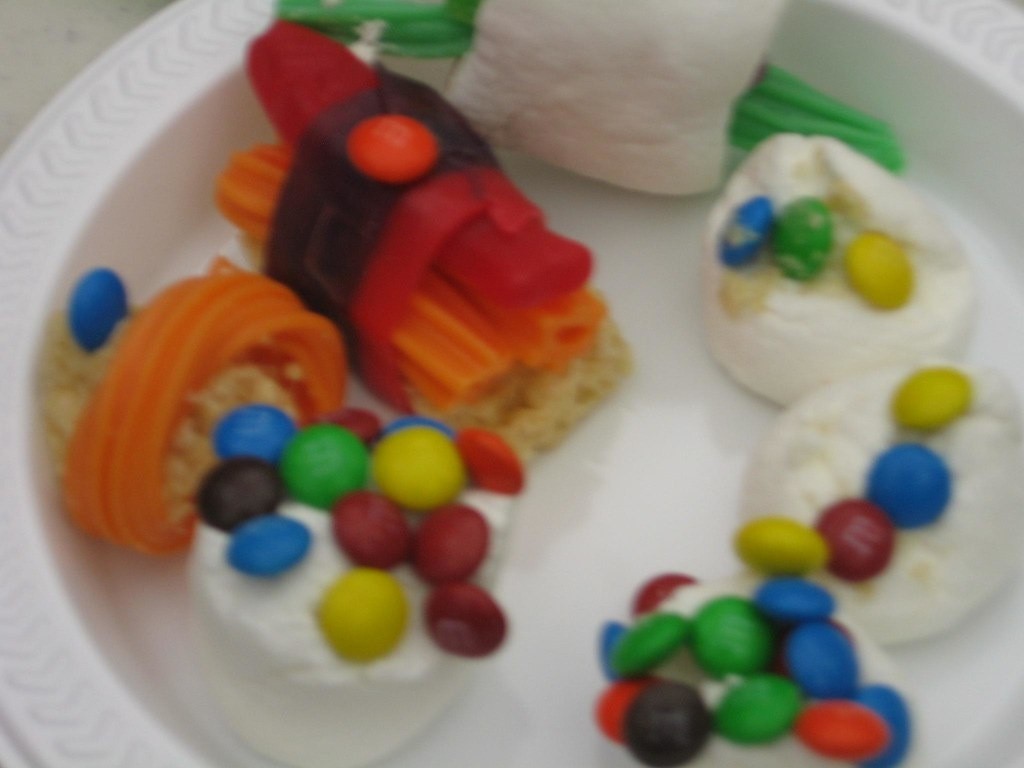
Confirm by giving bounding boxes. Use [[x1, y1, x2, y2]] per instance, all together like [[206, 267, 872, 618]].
[[40, 0, 1024, 768]]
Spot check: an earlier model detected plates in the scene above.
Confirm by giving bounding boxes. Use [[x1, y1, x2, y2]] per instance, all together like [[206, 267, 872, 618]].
[[0, 1, 1023, 768]]
